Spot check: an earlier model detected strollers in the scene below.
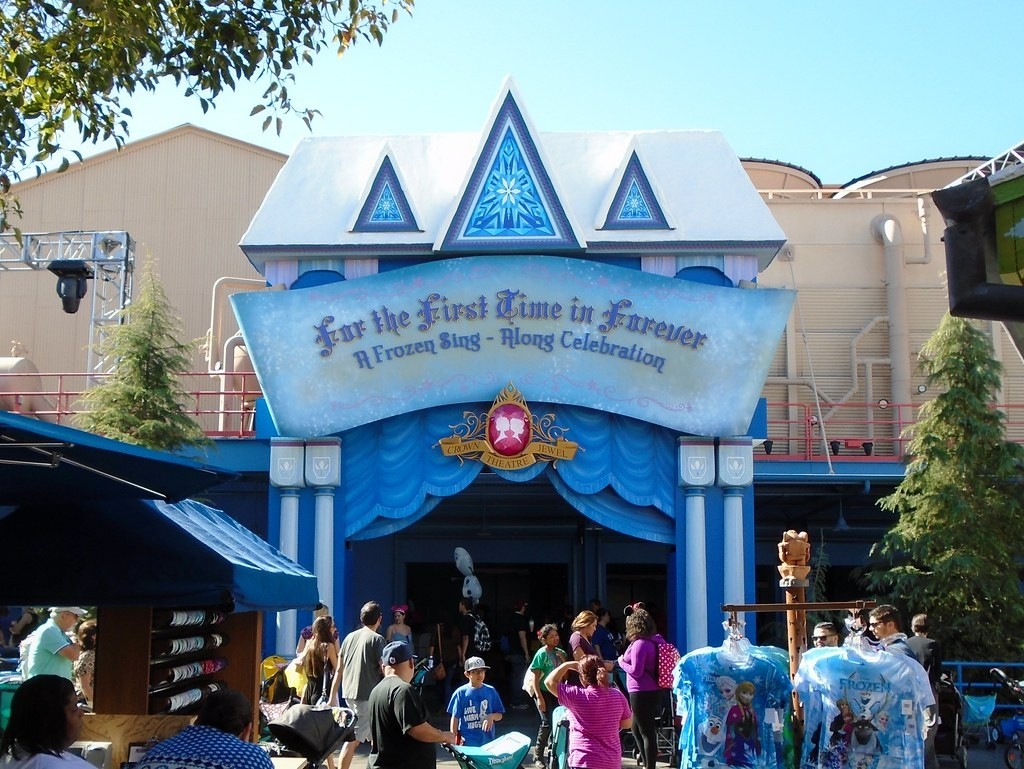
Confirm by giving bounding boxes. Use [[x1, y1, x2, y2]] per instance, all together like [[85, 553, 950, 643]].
[[266, 703, 358, 769], [547, 705, 570, 769], [440, 731, 531, 769], [990, 668, 1024, 769], [412, 656, 430, 696], [959, 673, 997, 751], [259, 655, 302, 741], [631, 690, 681, 767], [936, 678, 968, 769]]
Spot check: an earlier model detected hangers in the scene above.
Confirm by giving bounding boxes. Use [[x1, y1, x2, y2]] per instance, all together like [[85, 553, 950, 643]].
[[841, 619, 907, 662], [718, 605, 752, 664]]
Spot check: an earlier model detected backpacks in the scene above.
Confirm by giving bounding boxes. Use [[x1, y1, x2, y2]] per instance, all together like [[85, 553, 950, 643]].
[[465, 611, 492, 652], [629, 636, 681, 688]]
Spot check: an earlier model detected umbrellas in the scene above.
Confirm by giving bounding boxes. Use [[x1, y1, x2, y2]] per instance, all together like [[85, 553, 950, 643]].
[[0, 410, 241, 504]]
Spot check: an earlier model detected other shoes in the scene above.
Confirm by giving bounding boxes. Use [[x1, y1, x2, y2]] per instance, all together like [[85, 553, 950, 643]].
[[532, 754, 546, 769], [509, 703, 529, 710]]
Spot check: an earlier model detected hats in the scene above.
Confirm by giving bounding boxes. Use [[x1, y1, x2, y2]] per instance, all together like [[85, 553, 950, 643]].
[[464, 656, 491, 672], [195, 689, 252, 730], [382, 640, 418, 665], [360, 600, 383, 625], [47, 606, 88, 614]]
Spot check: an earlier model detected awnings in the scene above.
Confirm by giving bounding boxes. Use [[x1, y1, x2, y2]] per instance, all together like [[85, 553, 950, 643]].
[[139, 497, 321, 612]]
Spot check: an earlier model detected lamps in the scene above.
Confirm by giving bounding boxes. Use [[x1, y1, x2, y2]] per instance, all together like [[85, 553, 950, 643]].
[[763, 440, 773, 455], [47, 260, 99, 313], [862, 442, 873, 456], [830, 440, 840, 456]]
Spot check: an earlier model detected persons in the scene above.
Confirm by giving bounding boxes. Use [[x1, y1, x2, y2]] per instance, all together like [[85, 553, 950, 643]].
[[384, 603, 414, 654], [457, 599, 481, 688], [811, 621, 837, 647], [329, 601, 389, 769], [299, 617, 338, 706], [543, 655, 632, 769], [133, 690, 275, 768], [868, 604, 914, 659], [27, 606, 81, 681], [0, 674, 98, 769], [0, 606, 37, 671], [296, 604, 342, 656], [366, 641, 463, 769], [446, 656, 505, 747], [508, 598, 667, 769], [74, 618, 98, 709], [909, 613, 944, 769]]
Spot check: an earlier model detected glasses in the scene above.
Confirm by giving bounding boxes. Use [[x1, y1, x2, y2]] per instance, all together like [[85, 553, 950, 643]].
[[64, 611, 79, 621], [869, 622, 889, 628], [470, 670, 487, 674], [812, 635, 835, 640]]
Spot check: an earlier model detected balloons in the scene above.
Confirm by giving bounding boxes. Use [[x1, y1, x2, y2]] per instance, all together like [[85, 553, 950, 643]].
[[453, 546, 482, 600]]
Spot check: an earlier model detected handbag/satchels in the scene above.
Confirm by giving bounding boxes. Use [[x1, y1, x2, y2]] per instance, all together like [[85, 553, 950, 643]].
[[522, 649, 545, 697], [432, 663, 446, 681], [316, 694, 329, 705]]
